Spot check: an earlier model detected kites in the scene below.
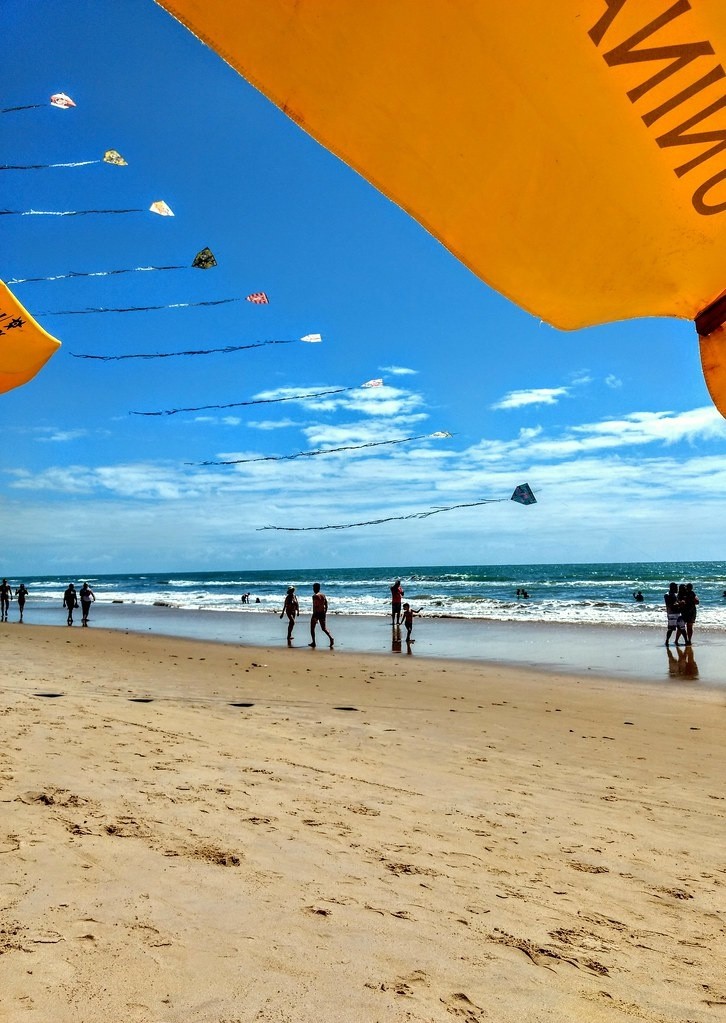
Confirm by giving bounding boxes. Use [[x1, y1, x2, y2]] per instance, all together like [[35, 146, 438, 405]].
[[0, 200, 175, 217], [127, 378, 538, 532], [6, 247, 321, 361], [0, 149, 129, 169], [0, 92, 77, 113]]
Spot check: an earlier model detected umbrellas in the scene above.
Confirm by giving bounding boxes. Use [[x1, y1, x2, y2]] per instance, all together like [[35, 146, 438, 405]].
[[160, 1, 725, 416]]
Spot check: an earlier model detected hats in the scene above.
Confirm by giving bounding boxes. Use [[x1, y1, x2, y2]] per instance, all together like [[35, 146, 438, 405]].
[[287, 586, 296, 594]]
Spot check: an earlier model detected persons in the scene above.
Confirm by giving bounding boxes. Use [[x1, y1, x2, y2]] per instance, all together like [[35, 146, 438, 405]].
[[15, 584, 28, 620], [398, 603, 423, 642], [256, 598, 260, 603], [242, 592, 250, 604], [279, 586, 300, 640], [0, 578, 13, 617], [79, 582, 96, 619], [723, 591, 726, 597], [664, 582, 699, 647], [63, 584, 78, 620], [390, 580, 404, 625], [307, 583, 335, 647], [517, 589, 529, 599], [633, 591, 644, 601]]
